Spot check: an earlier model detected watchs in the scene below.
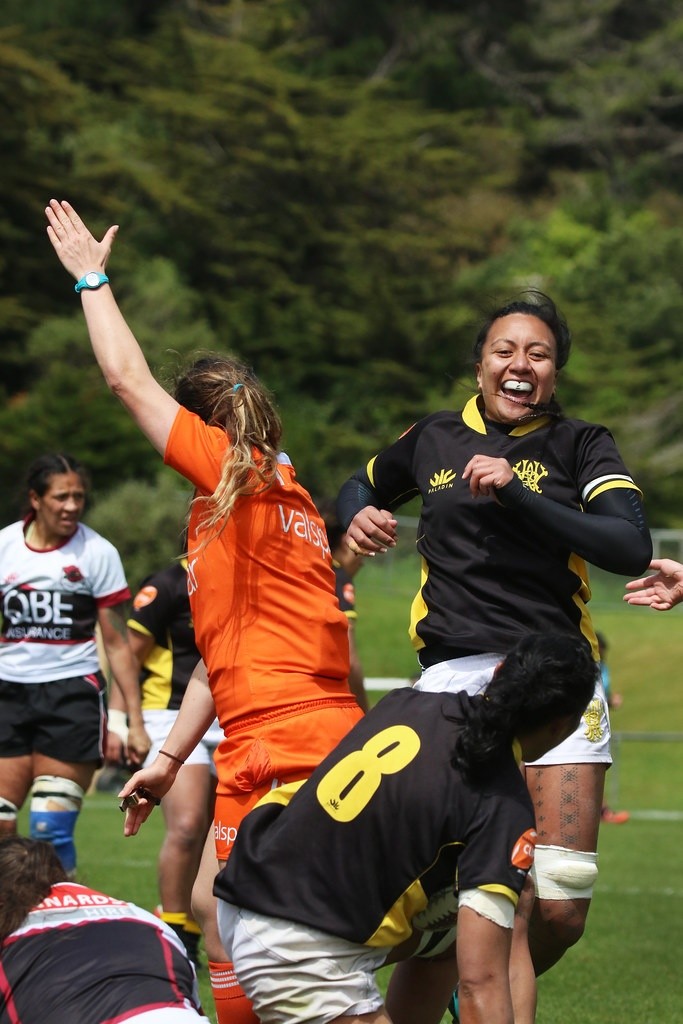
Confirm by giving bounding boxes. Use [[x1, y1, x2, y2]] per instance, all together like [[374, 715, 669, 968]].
[[75, 271, 109, 294]]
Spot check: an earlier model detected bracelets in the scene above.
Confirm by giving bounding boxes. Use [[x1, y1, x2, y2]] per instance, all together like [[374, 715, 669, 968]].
[[158, 750, 184, 765]]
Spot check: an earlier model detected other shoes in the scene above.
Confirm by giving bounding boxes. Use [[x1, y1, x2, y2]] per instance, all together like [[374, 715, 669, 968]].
[[603, 804, 628, 823]]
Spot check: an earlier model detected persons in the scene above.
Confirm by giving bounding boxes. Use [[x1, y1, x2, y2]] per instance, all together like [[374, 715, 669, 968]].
[[45, 199, 371, 1024], [0, 291, 682, 1024]]
